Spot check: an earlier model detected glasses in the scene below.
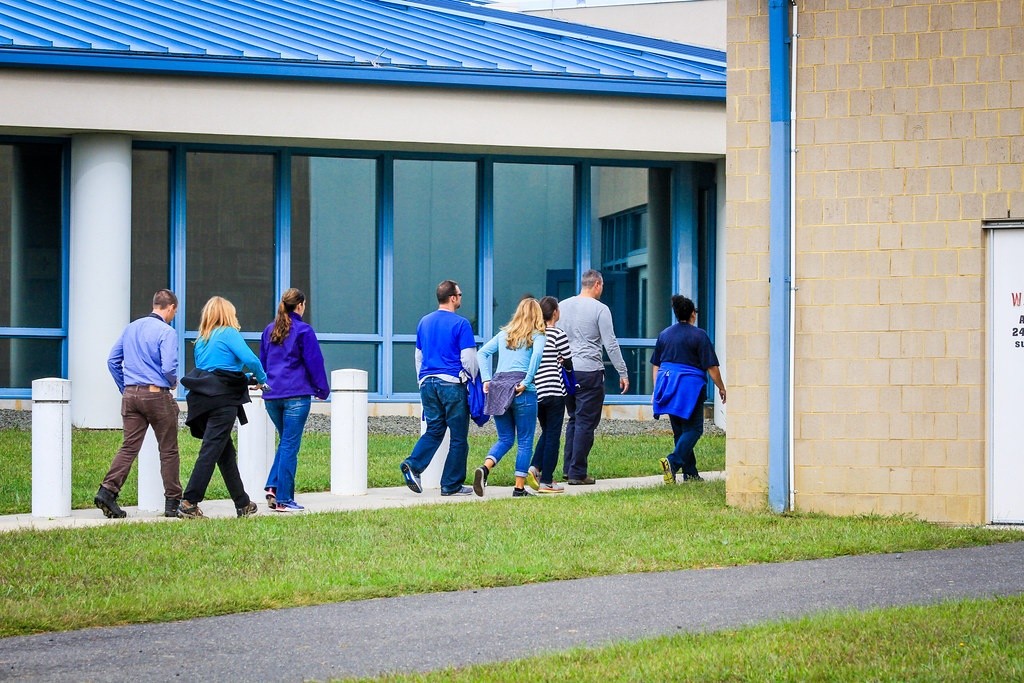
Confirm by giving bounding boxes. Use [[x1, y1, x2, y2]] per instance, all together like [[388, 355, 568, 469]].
[[693, 307, 698, 313], [452, 293, 462, 296]]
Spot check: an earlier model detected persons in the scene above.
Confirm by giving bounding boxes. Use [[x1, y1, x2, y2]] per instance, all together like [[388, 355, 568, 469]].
[[93, 289, 184, 518], [401, 280, 477, 496], [176, 296, 267, 519], [473, 298, 547, 497], [526, 296, 573, 493], [555, 270, 629, 485], [259, 289, 330, 511], [651, 295, 726, 485]]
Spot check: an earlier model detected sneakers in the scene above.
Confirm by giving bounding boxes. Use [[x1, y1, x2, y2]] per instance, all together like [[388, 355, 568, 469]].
[[538, 480, 564, 493], [568, 476, 595, 485], [400, 462, 423, 493], [275, 500, 304, 511], [265, 487, 277, 509], [236, 501, 257, 517], [473, 465, 489, 496], [177, 500, 209, 518], [94, 486, 126, 518], [441, 486, 473, 496], [512, 490, 536, 497], [684, 476, 704, 483], [658, 457, 676, 485], [562, 475, 570, 482], [525, 466, 540, 491], [165, 499, 180, 517]]
